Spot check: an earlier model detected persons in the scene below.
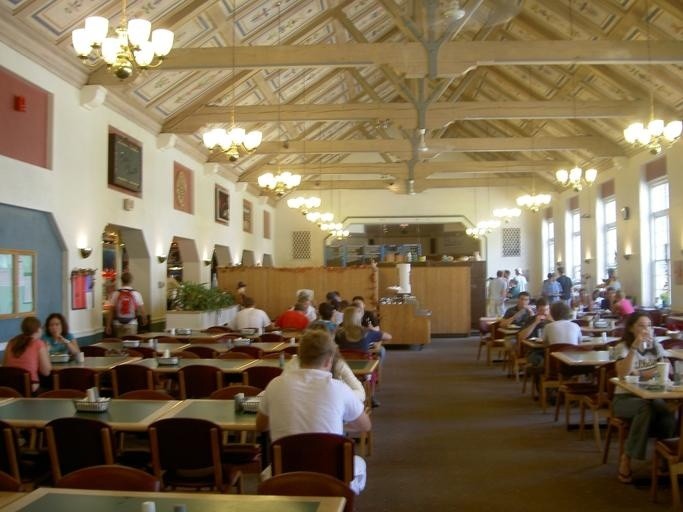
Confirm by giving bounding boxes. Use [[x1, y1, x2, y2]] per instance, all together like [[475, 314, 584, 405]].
[[41, 314, 81, 358], [610, 311, 678, 485], [485, 261, 634, 403], [4, 317, 51, 396], [104, 270, 393, 408], [253, 334, 371, 494]]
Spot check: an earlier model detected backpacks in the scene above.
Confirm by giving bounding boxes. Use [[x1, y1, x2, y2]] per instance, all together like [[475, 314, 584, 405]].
[[115, 289, 138, 325]]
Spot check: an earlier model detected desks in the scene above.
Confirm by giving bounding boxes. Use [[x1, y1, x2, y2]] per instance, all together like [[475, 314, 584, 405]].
[[380, 301, 432, 351], [0, 486, 348, 510], [1, 396, 374, 466]]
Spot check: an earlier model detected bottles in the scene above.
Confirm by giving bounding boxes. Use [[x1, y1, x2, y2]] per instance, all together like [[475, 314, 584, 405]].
[[147, 338, 153, 347], [376, 295, 416, 305], [163, 349, 170, 358], [278, 351, 284, 368], [291, 354, 297, 360], [290, 337, 295, 345]]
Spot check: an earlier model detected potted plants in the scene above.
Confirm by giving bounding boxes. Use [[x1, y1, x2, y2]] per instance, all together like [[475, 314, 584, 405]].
[[164, 281, 240, 332]]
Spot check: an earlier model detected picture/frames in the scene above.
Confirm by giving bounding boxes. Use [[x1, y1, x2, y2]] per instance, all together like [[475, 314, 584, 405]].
[[215, 188, 230, 223], [109, 132, 143, 194]]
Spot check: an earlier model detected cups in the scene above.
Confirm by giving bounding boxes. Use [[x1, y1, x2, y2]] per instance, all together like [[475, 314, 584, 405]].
[[225, 338, 232, 347], [640, 327, 654, 350], [75, 351, 84, 363], [152, 339, 158, 348], [655, 362, 670, 386], [174, 502, 187, 511], [141, 501, 155, 511], [232, 392, 244, 413], [674, 361, 683, 386], [366, 319, 373, 328], [86, 389, 94, 403], [91, 386, 98, 399], [169, 328, 175, 336], [536, 300, 682, 352], [624, 375, 640, 385]]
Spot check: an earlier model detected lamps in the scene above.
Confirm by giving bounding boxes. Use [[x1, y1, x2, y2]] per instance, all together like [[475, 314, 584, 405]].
[[554, 1, 596, 193], [256, 3, 301, 196], [623, 247, 630, 260], [625, 1, 683, 159], [77, 235, 93, 258], [461, 125, 522, 239], [203, 257, 212, 266], [72, 1, 176, 81], [515, 65, 552, 213], [157, 250, 166, 263], [310, 158, 351, 240], [202, 1, 261, 161], [287, 122, 322, 214], [584, 251, 592, 264]]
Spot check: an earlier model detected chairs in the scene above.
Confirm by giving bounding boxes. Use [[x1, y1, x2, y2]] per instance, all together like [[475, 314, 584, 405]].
[[0, 420, 50, 485], [46, 417, 155, 481], [268, 433, 355, 483], [146, 417, 246, 494], [0, 323, 378, 449], [478, 308, 680, 511], [256, 471, 358, 510], [56, 464, 161, 492]]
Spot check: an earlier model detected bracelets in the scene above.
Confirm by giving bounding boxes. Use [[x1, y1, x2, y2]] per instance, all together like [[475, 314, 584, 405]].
[[630, 344, 637, 353]]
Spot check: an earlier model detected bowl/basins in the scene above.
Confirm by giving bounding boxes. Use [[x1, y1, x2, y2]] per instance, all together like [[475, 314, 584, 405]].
[[242, 396, 263, 413]]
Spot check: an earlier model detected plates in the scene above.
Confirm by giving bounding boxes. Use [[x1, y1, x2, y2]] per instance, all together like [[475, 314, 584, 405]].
[[646, 378, 674, 387]]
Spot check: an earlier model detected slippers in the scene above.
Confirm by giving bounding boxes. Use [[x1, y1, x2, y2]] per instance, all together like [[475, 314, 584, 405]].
[[619, 469, 634, 485]]
